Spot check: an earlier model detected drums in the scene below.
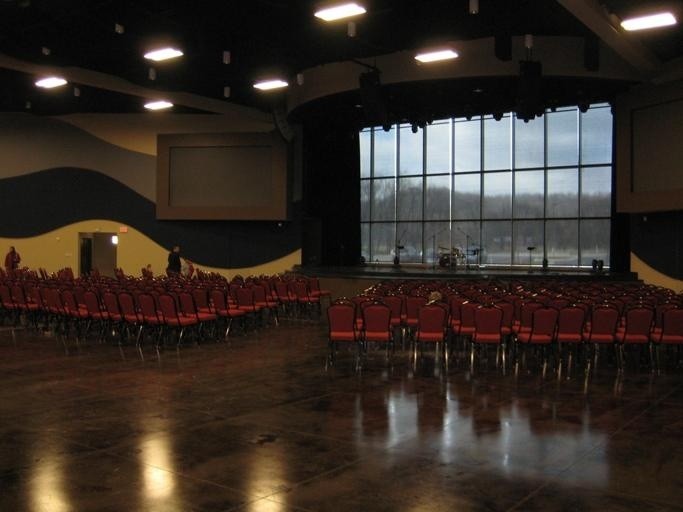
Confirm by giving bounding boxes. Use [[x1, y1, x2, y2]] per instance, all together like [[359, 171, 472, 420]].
[[440, 254, 455, 269]]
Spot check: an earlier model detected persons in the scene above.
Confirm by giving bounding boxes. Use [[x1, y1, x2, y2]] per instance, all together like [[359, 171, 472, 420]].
[[184, 257, 196, 278], [427, 290, 442, 304], [166, 244, 182, 278], [4, 246, 22, 276]]
